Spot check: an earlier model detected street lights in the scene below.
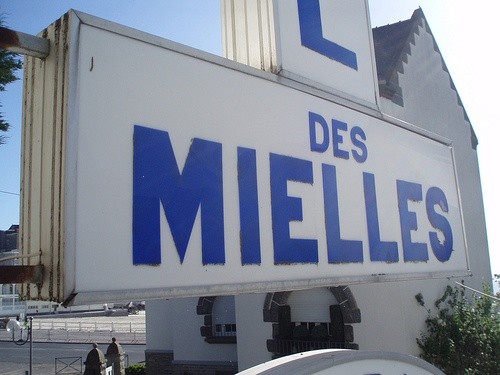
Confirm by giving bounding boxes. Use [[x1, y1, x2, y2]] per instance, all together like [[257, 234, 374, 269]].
[[6, 315, 33, 375]]
